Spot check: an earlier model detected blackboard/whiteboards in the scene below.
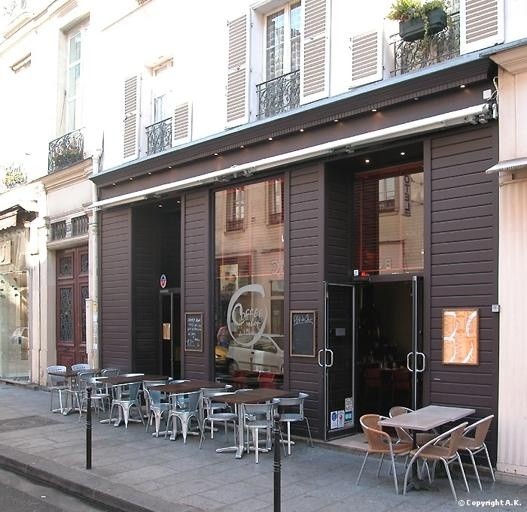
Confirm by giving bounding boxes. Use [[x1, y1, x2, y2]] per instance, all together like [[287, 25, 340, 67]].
[[184, 312, 204, 353], [290, 310, 316, 359]]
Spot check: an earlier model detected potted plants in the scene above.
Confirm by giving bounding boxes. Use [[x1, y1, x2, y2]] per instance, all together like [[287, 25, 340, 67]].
[[386, 1, 448, 49]]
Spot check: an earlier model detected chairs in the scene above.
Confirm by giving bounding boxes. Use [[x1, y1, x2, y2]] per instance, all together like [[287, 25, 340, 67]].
[[355, 406, 496, 501], [45, 362, 314, 464]]
